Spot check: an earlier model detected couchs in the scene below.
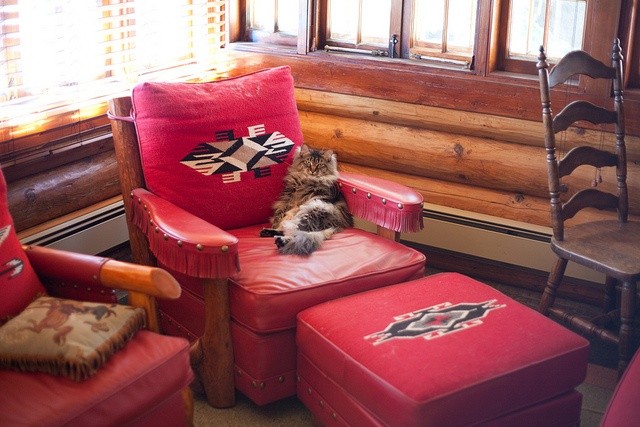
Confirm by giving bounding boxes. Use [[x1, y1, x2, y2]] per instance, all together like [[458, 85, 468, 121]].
[[294, 269, 594, 426], [0, 166, 197, 427], [105, 63, 429, 410]]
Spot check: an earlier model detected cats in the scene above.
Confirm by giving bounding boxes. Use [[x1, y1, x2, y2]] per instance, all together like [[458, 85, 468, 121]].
[[259, 142, 355, 257]]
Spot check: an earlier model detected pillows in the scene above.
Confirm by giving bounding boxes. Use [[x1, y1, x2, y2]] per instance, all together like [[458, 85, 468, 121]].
[[0, 292, 149, 383]]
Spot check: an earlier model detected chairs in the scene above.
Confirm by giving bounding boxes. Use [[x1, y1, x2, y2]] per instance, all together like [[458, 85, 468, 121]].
[[535, 36, 640, 384]]
